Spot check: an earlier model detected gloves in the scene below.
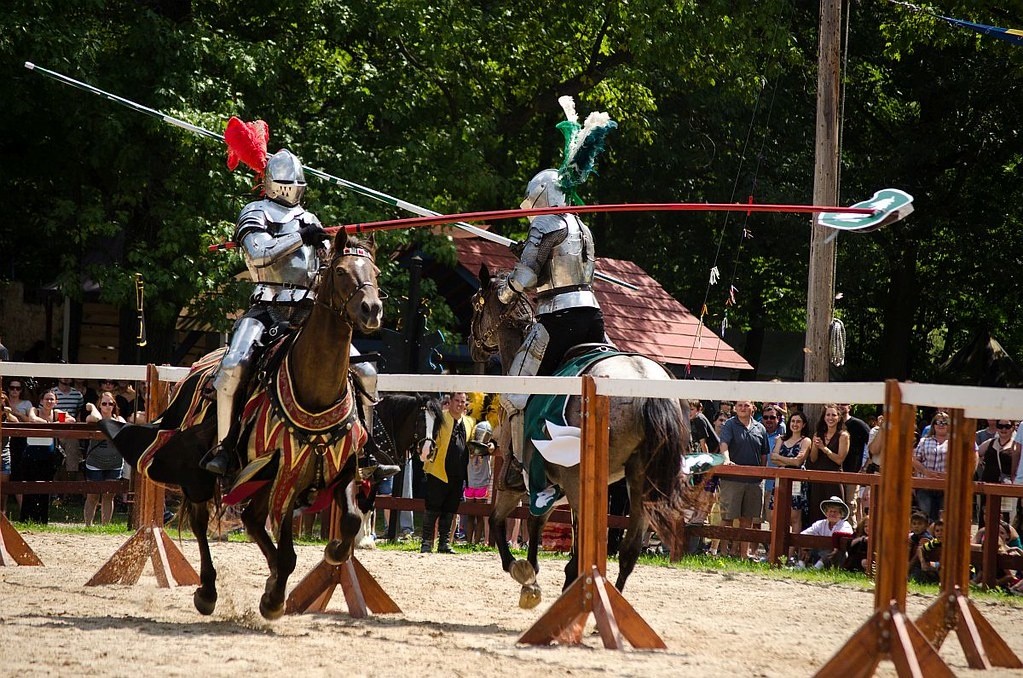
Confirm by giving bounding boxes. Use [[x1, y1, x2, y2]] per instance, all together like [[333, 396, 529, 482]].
[[297, 224, 328, 246]]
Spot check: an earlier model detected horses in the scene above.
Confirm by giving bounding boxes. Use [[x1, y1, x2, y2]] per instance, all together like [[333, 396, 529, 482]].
[[471, 261, 720, 609], [168, 224, 383, 621]]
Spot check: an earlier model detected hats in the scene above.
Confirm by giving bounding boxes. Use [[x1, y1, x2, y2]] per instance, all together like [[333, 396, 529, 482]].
[[820, 495, 850, 520]]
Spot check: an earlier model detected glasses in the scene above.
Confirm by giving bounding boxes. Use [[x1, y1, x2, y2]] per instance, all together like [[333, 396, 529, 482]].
[[935, 421, 948, 426], [763, 415, 776, 420], [9, 386, 21, 391], [997, 424, 1011, 429], [101, 402, 113, 406]]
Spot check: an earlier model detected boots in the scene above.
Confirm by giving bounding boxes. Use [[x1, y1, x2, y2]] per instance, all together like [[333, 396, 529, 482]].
[[421, 525, 433, 552], [438, 529, 457, 553]]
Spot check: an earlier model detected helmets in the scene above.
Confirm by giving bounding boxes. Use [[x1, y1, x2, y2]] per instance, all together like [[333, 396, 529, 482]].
[[466, 420, 496, 449], [223, 116, 307, 204], [519, 96, 618, 222]]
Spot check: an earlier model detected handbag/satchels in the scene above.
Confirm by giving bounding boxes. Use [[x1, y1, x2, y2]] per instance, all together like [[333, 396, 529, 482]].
[[54, 446, 66, 466]]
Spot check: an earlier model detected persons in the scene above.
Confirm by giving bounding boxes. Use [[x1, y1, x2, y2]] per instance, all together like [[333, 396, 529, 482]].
[[206, 149, 400, 486], [377, 389, 540, 556], [0, 376, 172, 527], [495, 168, 606, 517], [642, 400, 1023, 597]]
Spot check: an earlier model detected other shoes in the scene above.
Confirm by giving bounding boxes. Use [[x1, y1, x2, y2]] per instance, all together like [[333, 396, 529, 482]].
[[205, 452, 228, 473], [1010, 579, 1023, 589], [404, 533, 412, 540]]
